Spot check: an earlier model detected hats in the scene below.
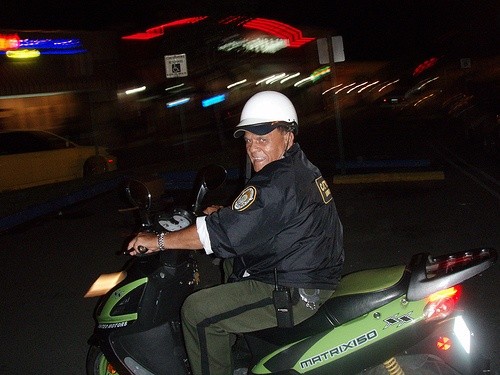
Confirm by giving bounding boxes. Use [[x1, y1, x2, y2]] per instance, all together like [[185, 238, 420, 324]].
[[234, 124, 299, 138]]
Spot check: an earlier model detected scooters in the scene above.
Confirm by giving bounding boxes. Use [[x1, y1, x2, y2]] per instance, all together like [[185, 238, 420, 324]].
[[85, 163, 500, 375]]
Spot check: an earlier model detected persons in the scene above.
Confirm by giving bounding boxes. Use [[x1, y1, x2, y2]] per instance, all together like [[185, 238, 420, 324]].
[[127, 90, 346, 375]]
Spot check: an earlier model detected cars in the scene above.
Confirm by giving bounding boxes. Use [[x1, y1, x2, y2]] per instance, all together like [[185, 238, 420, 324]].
[[0, 128, 120, 235]]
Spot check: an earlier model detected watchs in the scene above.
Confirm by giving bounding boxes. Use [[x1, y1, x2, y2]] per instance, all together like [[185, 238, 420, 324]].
[[156, 230, 169, 251]]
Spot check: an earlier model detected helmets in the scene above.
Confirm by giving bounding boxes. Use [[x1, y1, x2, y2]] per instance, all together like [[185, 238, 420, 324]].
[[235, 89, 298, 128]]
